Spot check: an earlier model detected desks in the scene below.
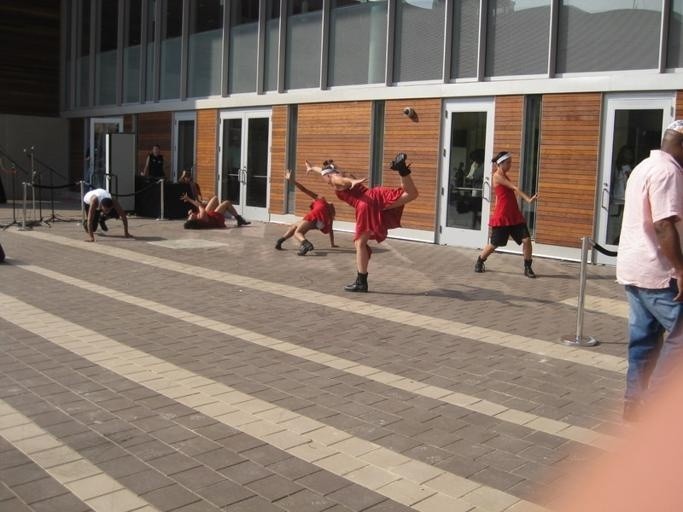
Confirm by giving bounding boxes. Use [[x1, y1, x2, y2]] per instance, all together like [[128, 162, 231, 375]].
[[135, 180, 194, 221]]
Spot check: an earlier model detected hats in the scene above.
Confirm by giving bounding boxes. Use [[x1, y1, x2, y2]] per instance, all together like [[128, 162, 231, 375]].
[[665, 119, 683, 135]]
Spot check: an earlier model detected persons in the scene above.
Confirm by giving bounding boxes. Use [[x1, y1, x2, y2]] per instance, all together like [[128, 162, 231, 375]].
[[80, 187, 135, 242], [137, 143, 171, 182], [176, 187, 252, 230], [304, 152, 418, 293], [612, 120, 683, 423], [273, 168, 341, 257], [0, 171, 7, 261], [472, 151, 541, 279], [453, 149, 481, 229], [611, 144, 634, 217], [226, 134, 241, 195]]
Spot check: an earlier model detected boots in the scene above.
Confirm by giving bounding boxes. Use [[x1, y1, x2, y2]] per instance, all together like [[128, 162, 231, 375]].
[[344, 272, 369, 293], [235, 215, 251, 226], [296, 239, 314, 257], [275, 236, 286, 250], [524, 259, 535, 278], [474, 255, 487, 273], [388, 152, 411, 177]]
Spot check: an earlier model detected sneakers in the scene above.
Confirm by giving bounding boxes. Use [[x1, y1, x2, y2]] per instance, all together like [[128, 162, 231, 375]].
[[623, 400, 643, 423], [98, 217, 108, 231]]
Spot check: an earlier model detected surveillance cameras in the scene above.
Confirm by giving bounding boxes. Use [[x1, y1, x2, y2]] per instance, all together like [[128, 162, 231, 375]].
[[403, 106, 414, 117]]
